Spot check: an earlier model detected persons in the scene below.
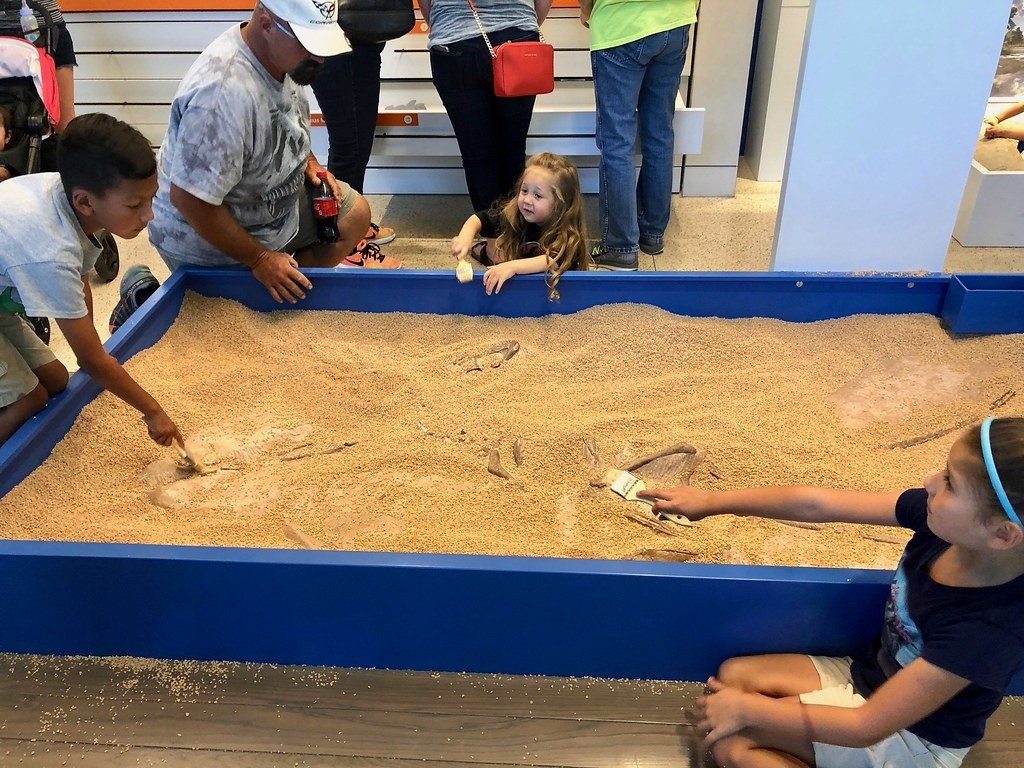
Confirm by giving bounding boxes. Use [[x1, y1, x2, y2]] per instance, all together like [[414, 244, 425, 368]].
[[0, 0, 120, 286], [451, 153, 592, 303], [0, 113, 188, 452], [310, 0, 402, 270], [983, 103, 1024, 154], [0, 105, 13, 182], [109, 1, 374, 336], [417, 0, 553, 268], [636, 418, 1024, 768], [578, 0, 701, 271]]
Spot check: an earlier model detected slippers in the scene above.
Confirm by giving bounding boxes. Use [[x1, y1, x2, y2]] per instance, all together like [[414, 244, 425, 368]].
[[471, 241, 492, 267]]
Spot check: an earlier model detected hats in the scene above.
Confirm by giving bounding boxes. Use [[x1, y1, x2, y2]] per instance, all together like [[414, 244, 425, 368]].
[[260, 0, 353, 57]]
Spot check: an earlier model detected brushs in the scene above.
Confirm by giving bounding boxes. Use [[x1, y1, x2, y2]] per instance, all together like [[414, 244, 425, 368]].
[[141, 415, 216, 476], [602, 466, 694, 529], [455, 252, 473, 282]]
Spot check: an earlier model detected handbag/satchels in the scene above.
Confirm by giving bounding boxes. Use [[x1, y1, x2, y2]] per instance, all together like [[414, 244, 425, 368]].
[[491, 42, 555, 96]]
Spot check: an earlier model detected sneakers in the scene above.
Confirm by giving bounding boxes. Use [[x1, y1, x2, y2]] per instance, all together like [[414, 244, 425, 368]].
[[638, 237, 664, 254], [359, 222, 396, 244], [334, 240, 402, 269], [109, 265, 160, 335], [588, 241, 638, 271]]
